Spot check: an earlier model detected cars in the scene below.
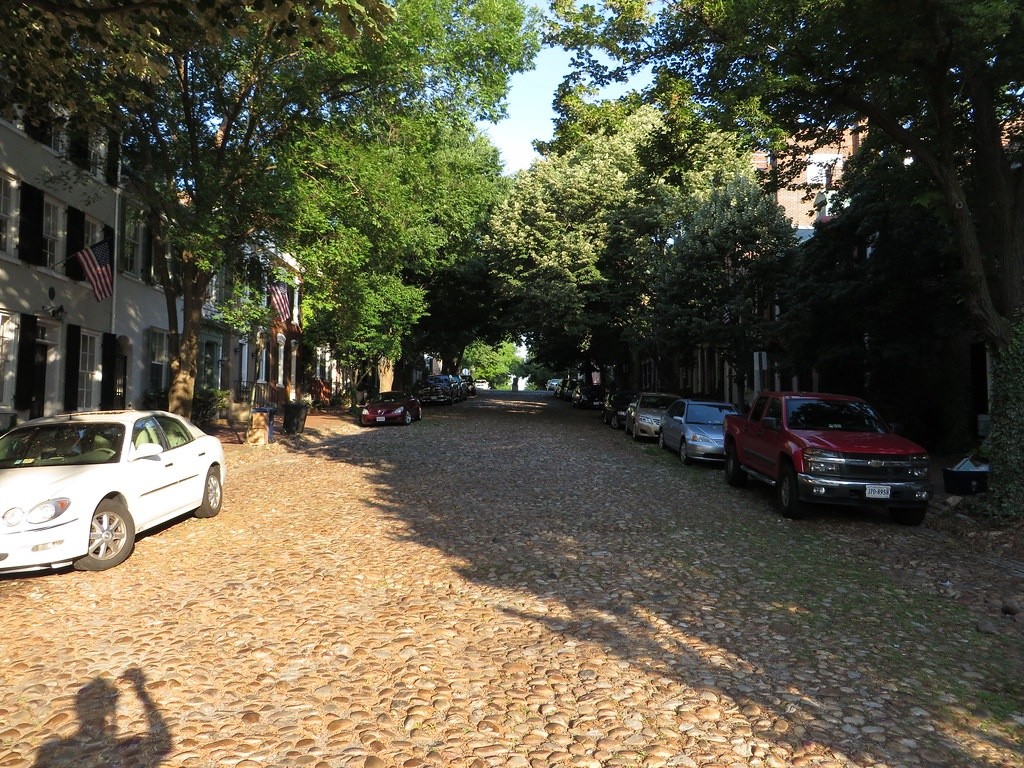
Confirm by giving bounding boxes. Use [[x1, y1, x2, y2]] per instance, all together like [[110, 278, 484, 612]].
[[359, 390, 424, 426], [417, 374, 491, 408], [624, 390, 682, 440], [0, 409, 227, 573], [658, 397, 746, 466], [545, 376, 645, 430]]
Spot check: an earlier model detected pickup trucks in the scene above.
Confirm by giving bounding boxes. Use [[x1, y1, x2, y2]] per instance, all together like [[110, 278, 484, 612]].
[[721, 390, 932, 528]]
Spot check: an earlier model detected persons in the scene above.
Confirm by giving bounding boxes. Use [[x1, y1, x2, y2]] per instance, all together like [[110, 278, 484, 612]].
[[453, 370, 460, 383]]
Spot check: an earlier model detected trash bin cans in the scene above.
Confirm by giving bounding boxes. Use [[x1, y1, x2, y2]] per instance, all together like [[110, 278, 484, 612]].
[[252, 407, 275, 444], [282, 402, 308, 433]]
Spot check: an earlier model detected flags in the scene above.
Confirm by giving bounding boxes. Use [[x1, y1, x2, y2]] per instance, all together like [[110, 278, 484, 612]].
[[264, 269, 290, 322], [78, 240, 113, 303]]
